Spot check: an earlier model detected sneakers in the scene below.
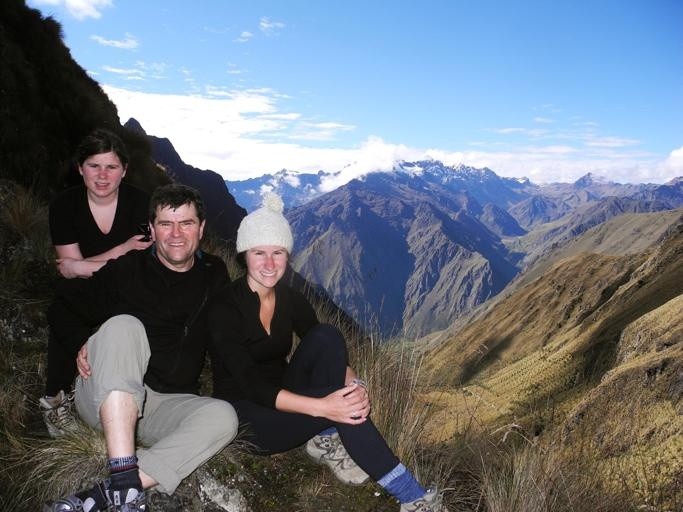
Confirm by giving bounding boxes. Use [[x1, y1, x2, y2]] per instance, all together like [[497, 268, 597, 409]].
[[305, 431, 372, 487], [51, 480, 113, 512], [37, 391, 82, 442], [399, 491, 451, 512], [112, 487, 150, 512]]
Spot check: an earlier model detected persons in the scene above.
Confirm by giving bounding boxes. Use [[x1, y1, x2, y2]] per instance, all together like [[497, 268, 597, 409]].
[[39, 130, 154, 409], [46, 183, 240, 512], [206, 192, 449, 511]]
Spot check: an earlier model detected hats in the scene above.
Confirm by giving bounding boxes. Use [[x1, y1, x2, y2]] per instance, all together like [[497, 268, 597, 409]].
[[236, 192, 295, 256]]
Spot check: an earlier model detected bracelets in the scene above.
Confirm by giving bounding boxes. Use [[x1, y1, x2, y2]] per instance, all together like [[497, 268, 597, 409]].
[[349, 379, 369, 395]]
